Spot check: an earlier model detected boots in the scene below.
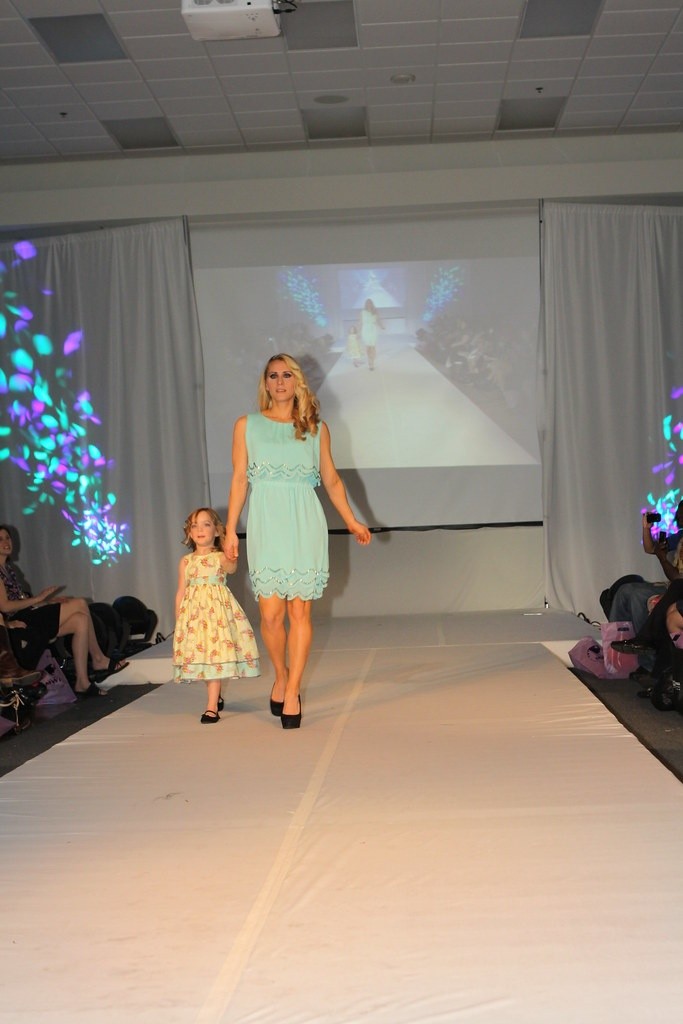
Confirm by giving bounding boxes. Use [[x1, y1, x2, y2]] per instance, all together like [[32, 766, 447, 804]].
[[0, 625, 41, 688]]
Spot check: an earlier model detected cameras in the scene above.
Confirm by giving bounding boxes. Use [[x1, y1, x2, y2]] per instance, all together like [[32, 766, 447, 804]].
[[647, 514, 661, 523]]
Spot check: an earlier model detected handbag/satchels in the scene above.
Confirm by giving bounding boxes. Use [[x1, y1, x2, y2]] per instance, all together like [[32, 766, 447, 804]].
[[600, 622, 640, 674], [568, 636, 631, 679], [36, 648, 77, 708]]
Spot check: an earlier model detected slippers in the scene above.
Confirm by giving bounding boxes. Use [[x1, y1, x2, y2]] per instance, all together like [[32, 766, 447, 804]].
[[94, 657, 129, 679], [74, 682, 107, 696]]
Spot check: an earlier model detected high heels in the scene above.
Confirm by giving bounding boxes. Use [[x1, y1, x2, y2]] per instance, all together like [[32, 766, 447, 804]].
[[270, 682, 284, 716], [281, 694, 301, 729]]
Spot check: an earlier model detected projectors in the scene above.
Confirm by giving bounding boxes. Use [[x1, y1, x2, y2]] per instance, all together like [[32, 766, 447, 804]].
[[181, 0, 281, 42]]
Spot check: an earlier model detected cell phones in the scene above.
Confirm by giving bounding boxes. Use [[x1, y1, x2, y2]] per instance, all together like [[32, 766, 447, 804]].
[[660, 531, 666, 545]]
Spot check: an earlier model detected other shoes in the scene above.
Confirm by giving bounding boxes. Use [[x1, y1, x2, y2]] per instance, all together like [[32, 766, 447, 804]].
[[637, 686, 654, 698], [218, 697, 224, 711], [201, 707, 217, 724], [611, 635, 656, 655]]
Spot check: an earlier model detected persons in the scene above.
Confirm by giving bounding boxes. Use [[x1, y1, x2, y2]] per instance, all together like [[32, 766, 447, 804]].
[[346, 326, 362, 367], [226, 353, 372, 729], [172, 508, 261, 724], [426, 318, 538, 402], [0, 526, 129, 697], [359, 298, 386, 371], [608, 501, 683, 699]]
